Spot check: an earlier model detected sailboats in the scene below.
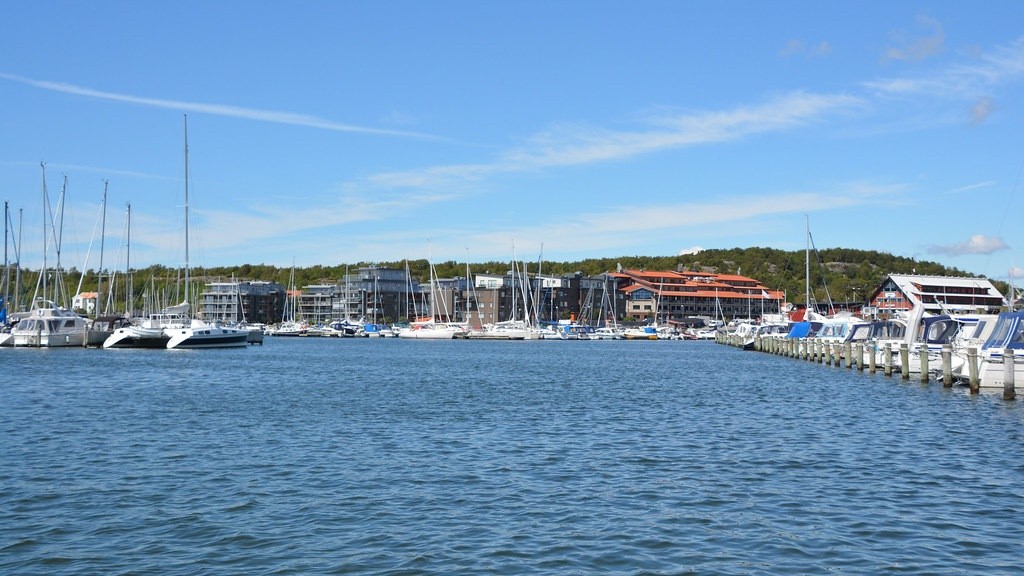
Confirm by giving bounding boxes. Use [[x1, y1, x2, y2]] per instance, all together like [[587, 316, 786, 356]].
[[0, 109, 1024, 393]]
